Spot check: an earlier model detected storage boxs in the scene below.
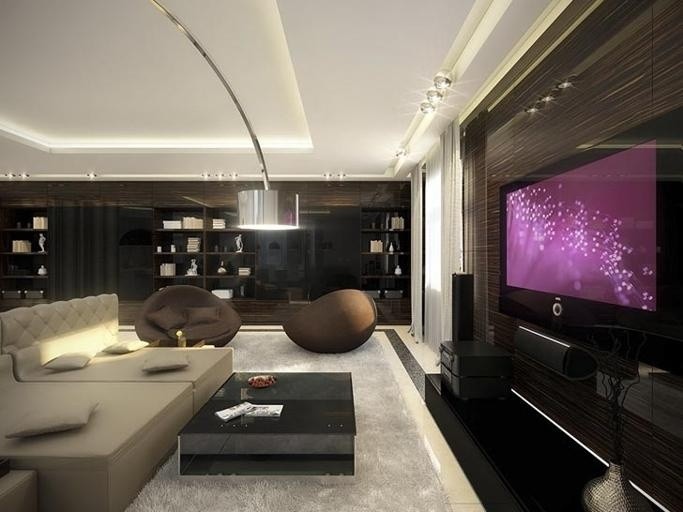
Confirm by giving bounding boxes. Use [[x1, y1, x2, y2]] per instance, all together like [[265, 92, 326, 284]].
[[440, 340, 512, 398]]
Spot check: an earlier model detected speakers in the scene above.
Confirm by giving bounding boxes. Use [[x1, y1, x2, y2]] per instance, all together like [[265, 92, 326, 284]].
[[454, 274, 474, 340]]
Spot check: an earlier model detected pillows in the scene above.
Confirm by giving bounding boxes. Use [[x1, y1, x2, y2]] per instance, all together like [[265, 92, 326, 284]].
[[141, 348, 188, 373], [145, 304, 185, 331], [43, 352, 91, 372], [186, 306, 220, 326], [5, 400, 99, 440], [101, 340, 148, 355]]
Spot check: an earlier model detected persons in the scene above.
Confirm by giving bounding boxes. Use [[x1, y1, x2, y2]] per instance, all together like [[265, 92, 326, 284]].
[[189, 259, 197, 275], [235, 234, 243, 251], [38, 234, 46, 252]]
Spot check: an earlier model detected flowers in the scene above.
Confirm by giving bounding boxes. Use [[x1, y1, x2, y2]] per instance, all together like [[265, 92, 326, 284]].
[[596, 364, 641, 464]]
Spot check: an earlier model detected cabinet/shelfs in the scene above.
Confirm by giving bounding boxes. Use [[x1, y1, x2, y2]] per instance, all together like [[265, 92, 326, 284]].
[[360, 207, 411, 299], [0, 207, 50, 299], [151, 206, 256, 299]]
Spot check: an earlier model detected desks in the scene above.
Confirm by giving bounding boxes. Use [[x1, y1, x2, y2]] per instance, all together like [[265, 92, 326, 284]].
[[425, 375, 667, 512], [0, 469, 37, 511]]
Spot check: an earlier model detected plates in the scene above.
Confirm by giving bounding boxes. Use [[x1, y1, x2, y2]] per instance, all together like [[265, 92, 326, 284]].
[[248, 375, 279, 389]]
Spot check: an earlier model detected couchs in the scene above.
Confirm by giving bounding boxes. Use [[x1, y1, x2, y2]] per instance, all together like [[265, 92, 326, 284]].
[[134, 285, 242, 347], [282, 290, 377, 354], [0, 292, 233, 512]]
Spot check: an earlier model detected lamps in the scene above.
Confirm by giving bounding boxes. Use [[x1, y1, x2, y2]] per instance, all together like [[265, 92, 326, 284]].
[[148, 1, 299, 232], [525, 103, 544, 113], [435, 70, 452, 89], [543, 92, 560, 105], [560, 77, 577, 92], [426, 89, 444, 102], [420, 103, 435, 114]]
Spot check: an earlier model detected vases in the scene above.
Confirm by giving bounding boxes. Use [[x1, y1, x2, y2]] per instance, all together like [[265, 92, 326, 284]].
[[583, 462, 639, 512]]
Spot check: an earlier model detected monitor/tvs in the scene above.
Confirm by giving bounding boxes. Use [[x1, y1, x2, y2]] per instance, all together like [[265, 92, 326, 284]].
[[498, 105, 683, 379]]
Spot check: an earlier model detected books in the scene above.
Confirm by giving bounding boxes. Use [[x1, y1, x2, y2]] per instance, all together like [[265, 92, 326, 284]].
[[212, 218, 226, 229], [32, 216, 47, 229], [164, 217, 201, 229], [213, 290, 233, 299], [160, 263, 176, 276], [370, 240, 383, 252], [187, 237, 201, 252], [391, 216, 404, 230], [244, 405, 284, 418], [12, 240, 31, 252], [238, 267, 251, 276], [215, 401, 256, 422]]
